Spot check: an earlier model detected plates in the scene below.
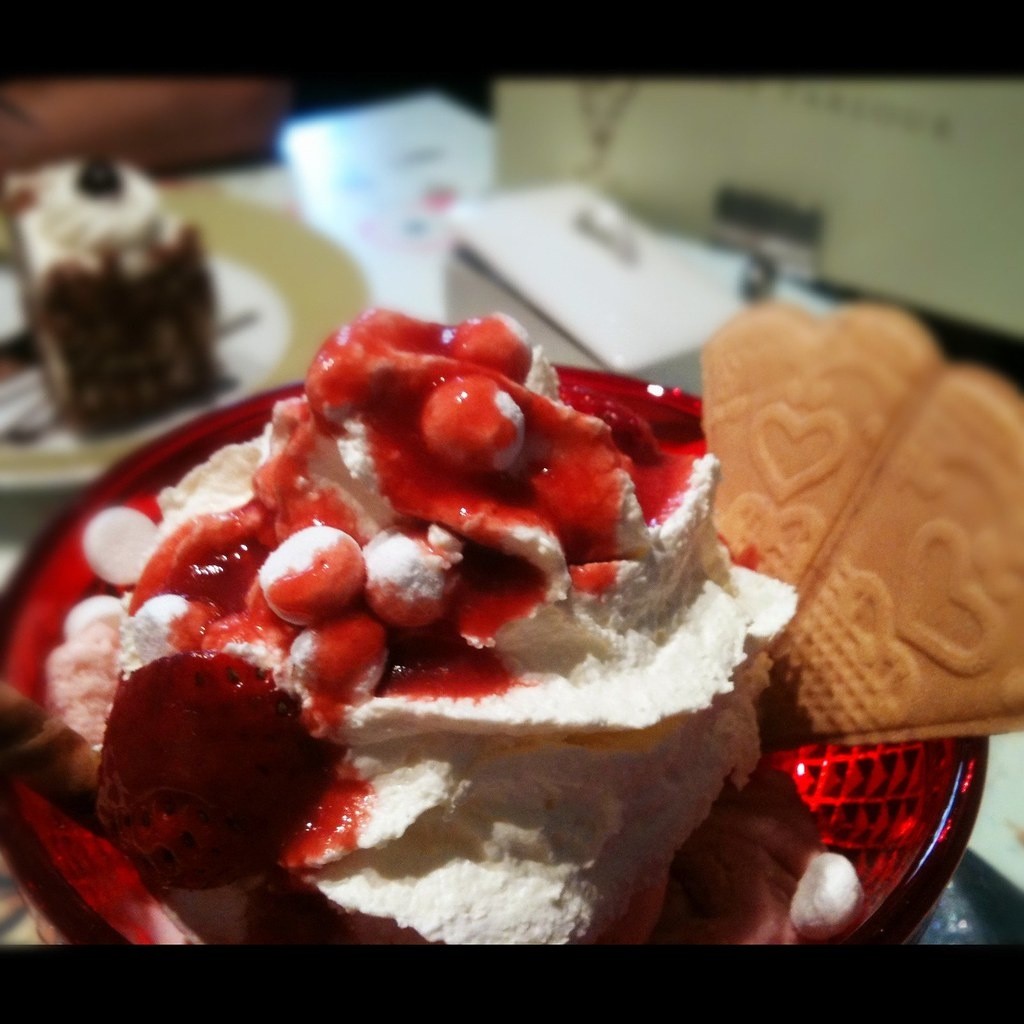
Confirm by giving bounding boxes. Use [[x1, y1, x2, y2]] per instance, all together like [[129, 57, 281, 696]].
[[0, 260, 293, 491]]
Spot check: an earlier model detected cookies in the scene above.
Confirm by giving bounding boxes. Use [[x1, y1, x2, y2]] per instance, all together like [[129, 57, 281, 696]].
[[692, 295, 1023, 750]]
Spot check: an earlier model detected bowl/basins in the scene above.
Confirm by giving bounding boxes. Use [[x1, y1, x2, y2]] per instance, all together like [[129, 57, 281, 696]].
[[0, 364, 987, 945]]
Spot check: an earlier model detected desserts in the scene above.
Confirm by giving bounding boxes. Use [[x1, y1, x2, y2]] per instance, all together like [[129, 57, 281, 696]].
[[43, 307, 799, 943], [12, 149, 219, 433]]
